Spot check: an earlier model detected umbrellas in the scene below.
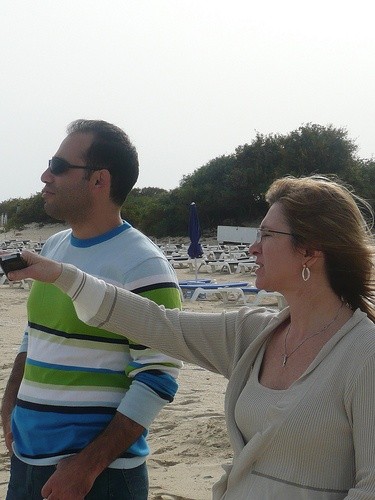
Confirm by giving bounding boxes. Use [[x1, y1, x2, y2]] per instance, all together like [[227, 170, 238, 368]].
[[187, 202, 204, 284]]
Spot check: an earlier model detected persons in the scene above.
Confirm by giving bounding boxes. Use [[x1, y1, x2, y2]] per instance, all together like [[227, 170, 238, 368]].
[[0, 119, 183, 500], [1, 174, 375, 500]]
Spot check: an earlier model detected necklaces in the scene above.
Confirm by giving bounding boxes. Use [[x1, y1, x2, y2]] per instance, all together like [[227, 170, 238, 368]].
[[282, 302, 344, 367]]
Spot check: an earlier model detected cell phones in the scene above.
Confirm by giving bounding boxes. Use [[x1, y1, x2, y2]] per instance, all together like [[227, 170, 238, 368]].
[[0, 252, 28, 281]]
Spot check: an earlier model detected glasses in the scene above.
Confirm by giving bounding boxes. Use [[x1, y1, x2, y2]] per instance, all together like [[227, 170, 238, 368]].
[[255, 228, 306, 245], [49, 160, 93, 175]]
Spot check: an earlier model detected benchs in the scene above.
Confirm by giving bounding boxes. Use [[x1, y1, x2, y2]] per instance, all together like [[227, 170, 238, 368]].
[[0, 238, 288, 310]]
